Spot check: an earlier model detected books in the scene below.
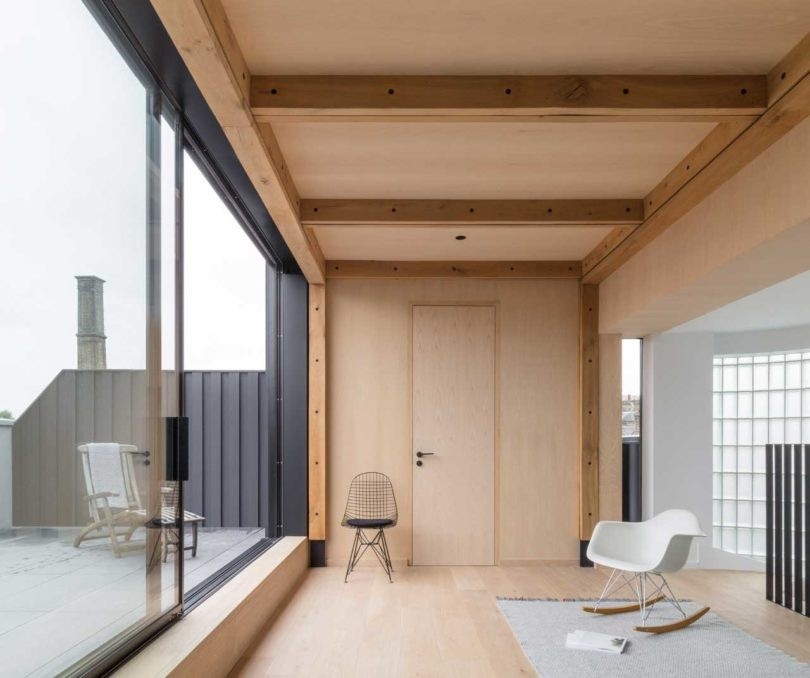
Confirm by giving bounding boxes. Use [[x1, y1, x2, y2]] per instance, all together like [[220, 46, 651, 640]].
[[565, 629, 627, 655]]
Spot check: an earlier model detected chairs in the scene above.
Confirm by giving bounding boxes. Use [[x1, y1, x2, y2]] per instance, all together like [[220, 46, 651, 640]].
[[73, 441, 206, 563], [583, 508, 711, 634], [340, 471, 398, 583]]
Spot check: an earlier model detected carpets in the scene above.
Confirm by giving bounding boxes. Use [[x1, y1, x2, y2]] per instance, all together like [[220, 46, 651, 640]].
[[495, 596, 810, 678]]
